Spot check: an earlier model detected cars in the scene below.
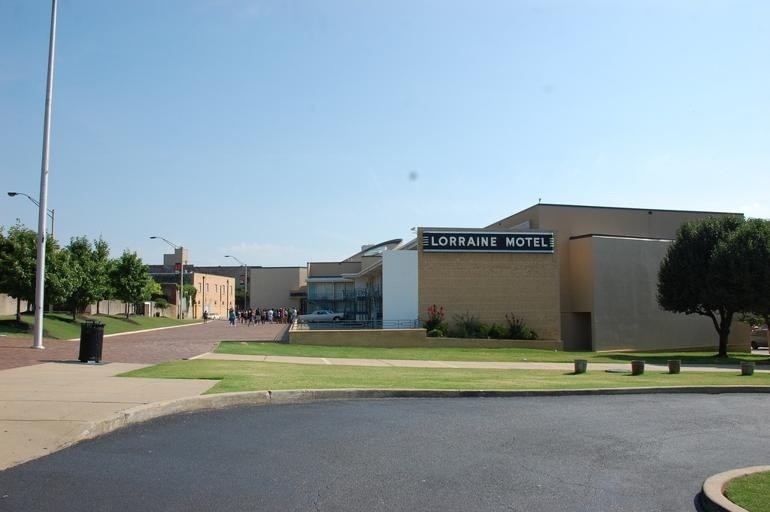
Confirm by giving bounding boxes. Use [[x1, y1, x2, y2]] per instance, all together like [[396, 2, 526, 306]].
[[298, 310, 345, 322], [207, 312, 223, 321], [751, 323, 769, 350]]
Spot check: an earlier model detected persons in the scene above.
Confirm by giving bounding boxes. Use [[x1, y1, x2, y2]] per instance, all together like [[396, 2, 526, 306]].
[[203, 311, 208, 324], [228, 307, 297, 327]]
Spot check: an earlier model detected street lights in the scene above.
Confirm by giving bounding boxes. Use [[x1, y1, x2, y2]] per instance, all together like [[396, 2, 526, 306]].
[[222, 255, 247, 312], [7, 190, 55, 241], [147, 236, 185, 321]]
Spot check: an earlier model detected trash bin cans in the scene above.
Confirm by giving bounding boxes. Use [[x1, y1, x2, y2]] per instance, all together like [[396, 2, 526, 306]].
[[79, 320, 106, 363]]
